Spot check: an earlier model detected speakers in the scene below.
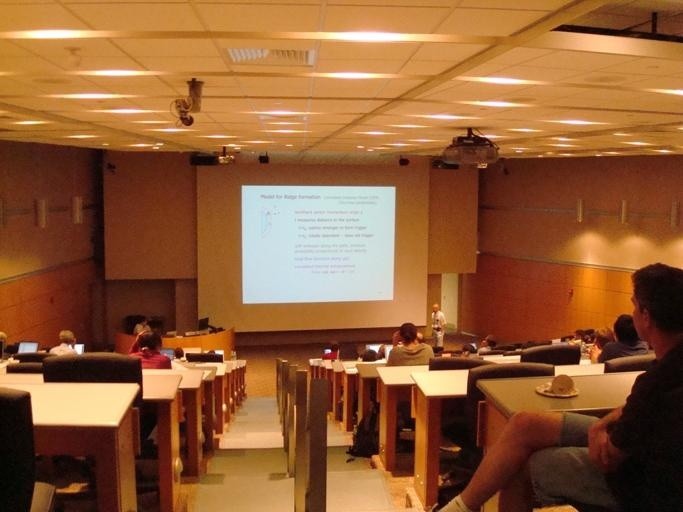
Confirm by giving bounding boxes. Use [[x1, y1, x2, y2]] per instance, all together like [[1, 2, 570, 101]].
[[399, 158, 409, 166], [189, 155, 219, 166], [259, 156, 269, 163], [432, 160, 460, 170]]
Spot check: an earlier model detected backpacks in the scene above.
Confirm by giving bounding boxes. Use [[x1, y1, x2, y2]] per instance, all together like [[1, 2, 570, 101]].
[[345, 403, 400, 459]]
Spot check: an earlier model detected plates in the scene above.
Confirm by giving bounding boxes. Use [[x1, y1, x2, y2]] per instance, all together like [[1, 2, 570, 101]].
[[534, 382, 579, 397]]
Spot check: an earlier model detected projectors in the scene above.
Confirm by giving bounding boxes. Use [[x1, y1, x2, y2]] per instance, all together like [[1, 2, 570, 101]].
[[442, 143, 500, 168]]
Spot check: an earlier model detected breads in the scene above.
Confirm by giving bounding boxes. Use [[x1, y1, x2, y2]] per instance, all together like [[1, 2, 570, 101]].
[[552, 374, 575, 395]]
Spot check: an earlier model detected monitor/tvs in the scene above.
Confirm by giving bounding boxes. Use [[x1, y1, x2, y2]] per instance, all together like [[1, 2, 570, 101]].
[[198, 317, 209, 330]]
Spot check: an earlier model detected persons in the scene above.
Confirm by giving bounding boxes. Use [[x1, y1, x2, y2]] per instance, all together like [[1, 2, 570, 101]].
[[320, 323, 435, 428], [567, 315, 650, 365], [438, 263, 683, 511], [127, 329, 171, 369], [0, 331, 7, 354], [170, 348, 187, 363], [476, 334, 499, 355], [133, 316, 151, 335], [48, 329, 78, 357], [431, 304, 447, 348]]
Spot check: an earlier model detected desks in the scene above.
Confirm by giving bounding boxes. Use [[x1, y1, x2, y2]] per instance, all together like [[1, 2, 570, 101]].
[[1, 346, 247, 512], [307, 335, 658, 512]]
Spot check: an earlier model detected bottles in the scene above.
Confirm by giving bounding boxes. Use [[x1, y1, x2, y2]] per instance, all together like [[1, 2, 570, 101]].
[[229, 346, 237, 363]]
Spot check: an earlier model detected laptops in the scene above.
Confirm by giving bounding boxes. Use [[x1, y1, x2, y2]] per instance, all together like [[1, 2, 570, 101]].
[[383, 343, 395, 358], [159, 347, 175, 360], [365, 342, 383, 355], [72, 343, 85, 356], [323, 349, 332, 355], [16, 341, 39, 355]]
